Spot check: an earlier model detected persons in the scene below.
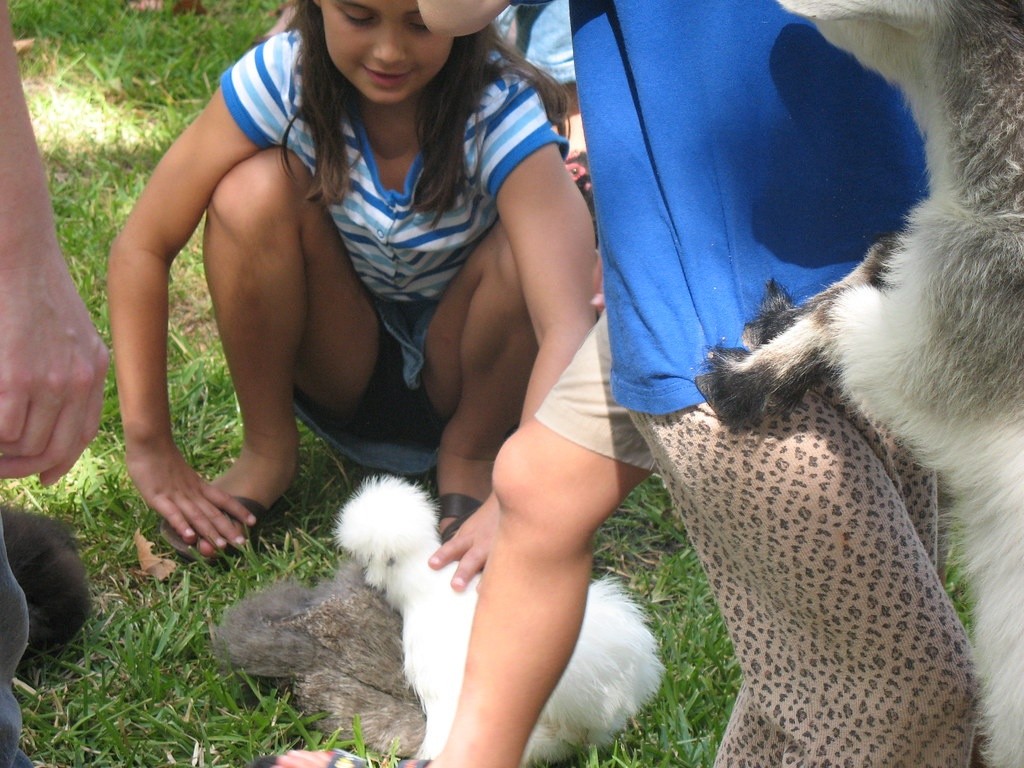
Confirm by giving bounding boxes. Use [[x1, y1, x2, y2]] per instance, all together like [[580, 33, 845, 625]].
[[251, 1, 1022, 768], [0, 1, 110, 489], [112, 1, 603, 591]]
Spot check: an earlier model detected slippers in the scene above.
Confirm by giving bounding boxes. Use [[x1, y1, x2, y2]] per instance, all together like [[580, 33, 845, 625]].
[[160, 489, 304, 563], [430, 469, 484, 570]]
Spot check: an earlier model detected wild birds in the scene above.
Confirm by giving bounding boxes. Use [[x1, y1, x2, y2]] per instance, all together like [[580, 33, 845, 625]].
[[3, 468, 666, 766]]
